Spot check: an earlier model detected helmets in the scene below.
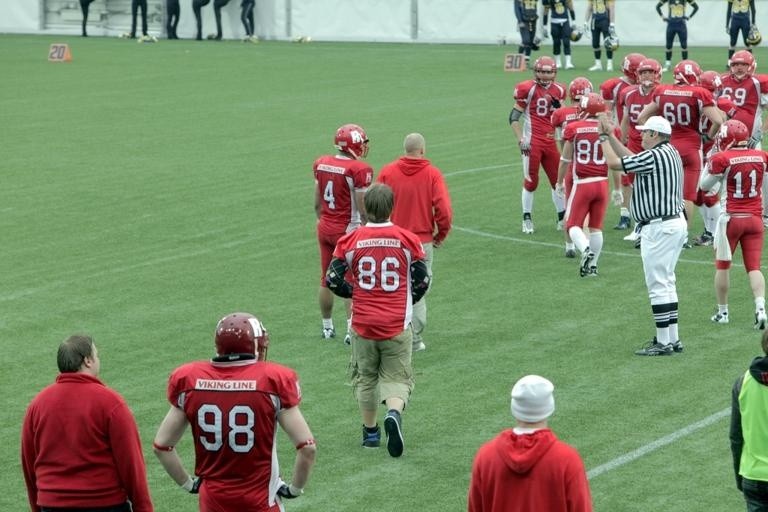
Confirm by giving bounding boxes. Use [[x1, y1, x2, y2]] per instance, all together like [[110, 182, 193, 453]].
[[334, 124, 370, 160], [622, 25, 762, 91], [567, 26, 619, 52], [533, 55, 556, 86], [717, 120, 748, 149], [570, 78, 605, 115], [215, 312, 270, 356]]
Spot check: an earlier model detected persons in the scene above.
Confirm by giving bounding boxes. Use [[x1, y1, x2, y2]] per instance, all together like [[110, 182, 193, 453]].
[[313, 124, 373, 343], [511, 52, 768, 356], [77, 1, 255, 41], [725, 331, 767, 512], [19, 336, 150, 511], [375, 133, 451, 351], [466, 375, 591, 512], [512, 0, 758, 72], [151, 314, 315, 512], [324, 185, 431, 458]]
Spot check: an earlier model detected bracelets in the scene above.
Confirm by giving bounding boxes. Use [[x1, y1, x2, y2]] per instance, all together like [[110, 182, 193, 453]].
[[181, 477, 193, 491], [289, 485, 302, 496], [297, 440, 314, 451], [152, 445, 174, 452]]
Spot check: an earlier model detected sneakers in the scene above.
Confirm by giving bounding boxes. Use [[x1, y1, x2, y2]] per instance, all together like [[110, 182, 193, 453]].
[[362, 423, 381, 449], [613, 216, 713, 248], [753, 308, 767, 330], [638, 337, 683, 357], [320, 328, 428, 351], [710, 310, 729, 324], [521, 219, 533, 234], [384, 412, 404, 458], [556, 219, 599, 278]]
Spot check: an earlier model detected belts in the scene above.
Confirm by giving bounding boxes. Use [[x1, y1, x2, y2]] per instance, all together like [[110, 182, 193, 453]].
[[642, 214, 681, 225]]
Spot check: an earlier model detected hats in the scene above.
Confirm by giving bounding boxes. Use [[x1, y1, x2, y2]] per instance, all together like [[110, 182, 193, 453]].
[[634, 116, 672, 135], [510, 374, 555, 423]]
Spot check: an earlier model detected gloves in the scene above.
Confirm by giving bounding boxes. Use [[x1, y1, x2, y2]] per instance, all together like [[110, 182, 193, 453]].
[[555, 183, 564, 200], [187, 478, 201, 494], [276, 484, 298, 500], [611, 190, 623, 206], [518, 139, 532, 156]]
